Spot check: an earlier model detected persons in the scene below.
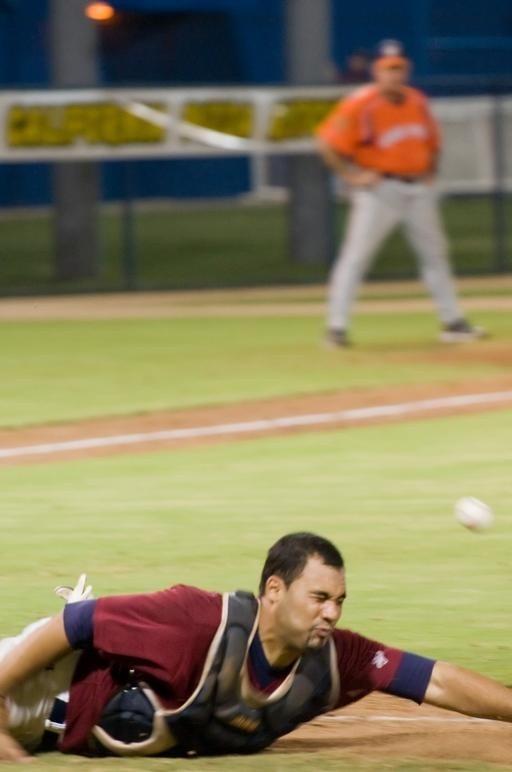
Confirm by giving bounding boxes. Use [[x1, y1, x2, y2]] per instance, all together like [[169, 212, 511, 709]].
[[313, 45, 490, 348], [1, 533, 511, 762]]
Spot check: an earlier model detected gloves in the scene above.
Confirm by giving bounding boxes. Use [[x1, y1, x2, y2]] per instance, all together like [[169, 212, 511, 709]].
[[56, 574, 93, 604]]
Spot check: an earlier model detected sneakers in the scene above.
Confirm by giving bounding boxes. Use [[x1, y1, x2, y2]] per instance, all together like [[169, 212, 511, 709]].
[[441, 322, 490, 343], [324, 328, 351, 352]]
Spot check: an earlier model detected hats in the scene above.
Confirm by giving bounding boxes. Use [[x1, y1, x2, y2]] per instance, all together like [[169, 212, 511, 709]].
[[372, 39, 411, 68]]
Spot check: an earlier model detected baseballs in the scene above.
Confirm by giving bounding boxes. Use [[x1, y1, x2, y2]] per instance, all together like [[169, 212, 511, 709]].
[[456, 496, 494, 531]]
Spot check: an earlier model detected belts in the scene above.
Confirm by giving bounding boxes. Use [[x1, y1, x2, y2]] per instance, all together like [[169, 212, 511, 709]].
[[41, 699, 67, 751], [383, 172, 414, 183]]
